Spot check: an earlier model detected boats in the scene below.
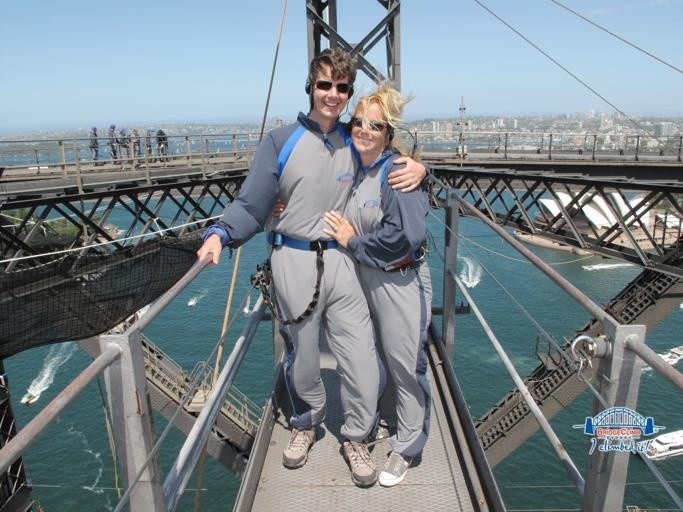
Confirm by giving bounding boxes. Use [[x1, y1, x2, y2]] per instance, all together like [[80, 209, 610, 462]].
[[24, 393, 35, 405], [669, 344, 682, 357], [637, 429, 683, 461], [187, 298, 197, 307], [624, 504, 639, 512]]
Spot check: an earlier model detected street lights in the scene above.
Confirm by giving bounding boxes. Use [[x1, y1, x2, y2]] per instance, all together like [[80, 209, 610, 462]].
[[456, 93, 467, 167]]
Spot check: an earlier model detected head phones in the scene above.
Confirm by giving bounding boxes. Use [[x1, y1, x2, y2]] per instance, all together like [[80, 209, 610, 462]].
[[305, 82, 354, 98]]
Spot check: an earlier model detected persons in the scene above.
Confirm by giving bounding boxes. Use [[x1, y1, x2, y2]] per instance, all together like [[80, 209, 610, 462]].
[[89, 125, 169, 166], [271, 82, 432, 487], [196, 47, 431, 488]]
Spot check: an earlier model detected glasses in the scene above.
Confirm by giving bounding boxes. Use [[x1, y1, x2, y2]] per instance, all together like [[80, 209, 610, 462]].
[[351, 117, 384, 131], [314, 80, 350, 93]]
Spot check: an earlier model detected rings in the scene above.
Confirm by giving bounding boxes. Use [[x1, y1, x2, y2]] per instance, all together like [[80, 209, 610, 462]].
[[334, 226, 338, 231]]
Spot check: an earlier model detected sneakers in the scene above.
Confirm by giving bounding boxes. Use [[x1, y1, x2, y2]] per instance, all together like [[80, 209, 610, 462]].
[[364, 422, 397, 446], [378, 450, 415, 487], [341, 436, 378, 488], [281, 425, 318, 469]]
